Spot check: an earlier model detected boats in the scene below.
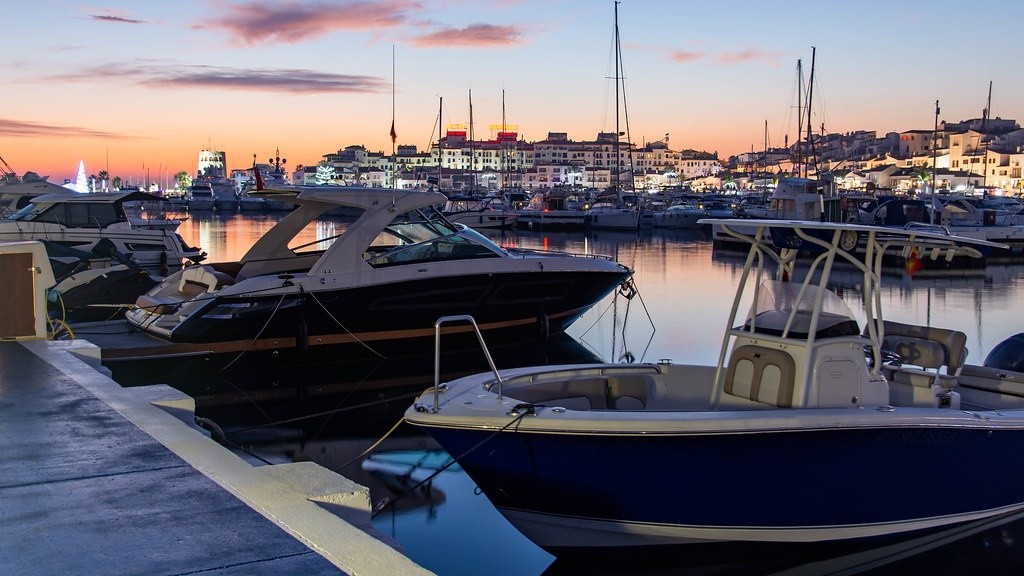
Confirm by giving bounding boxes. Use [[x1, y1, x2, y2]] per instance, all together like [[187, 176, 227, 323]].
[[0, 0, 1024, 278], [402, 217, 1023, 567], [56, 184, 635, 408]]
